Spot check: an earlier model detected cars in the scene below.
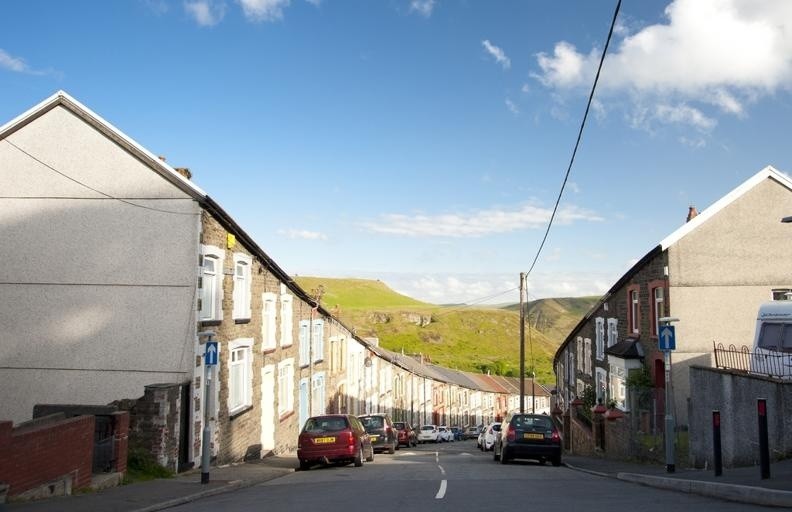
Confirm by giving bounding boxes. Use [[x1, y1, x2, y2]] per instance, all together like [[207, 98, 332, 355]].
[[390, 409, 566, 469], [357, 410, 398, 456], [294, 412, 376, 471]]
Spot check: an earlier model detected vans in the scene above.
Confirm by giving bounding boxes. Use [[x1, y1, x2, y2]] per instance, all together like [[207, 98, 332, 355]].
[[748, 297, 792, 381]]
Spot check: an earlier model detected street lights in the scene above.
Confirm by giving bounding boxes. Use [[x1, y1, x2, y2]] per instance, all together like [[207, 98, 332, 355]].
[[194, 328, 217, 484], [657, 313, 682, 474]]
